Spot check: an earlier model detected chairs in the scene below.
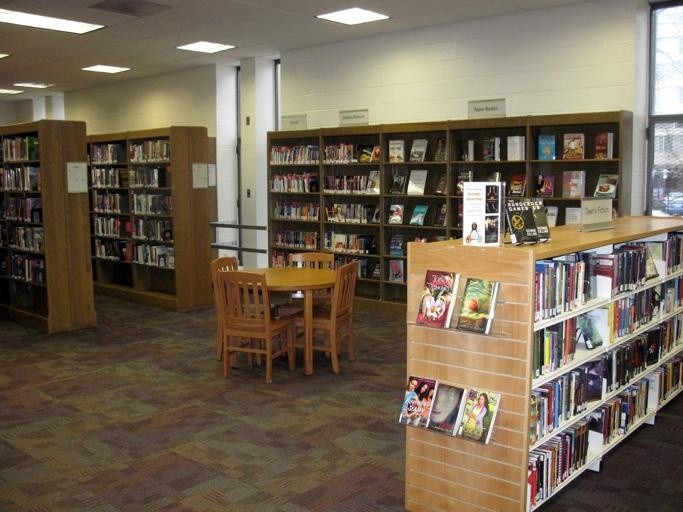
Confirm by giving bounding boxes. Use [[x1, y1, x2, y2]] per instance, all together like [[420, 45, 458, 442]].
[[207, 250, 360, 384]]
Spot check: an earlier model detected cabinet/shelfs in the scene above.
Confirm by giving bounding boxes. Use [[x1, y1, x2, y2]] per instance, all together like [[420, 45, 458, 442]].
[[86, 125, 217, 314], [516, 219, 682, 511], [0, 118, 98, 335], [266, 109, 634, 303]]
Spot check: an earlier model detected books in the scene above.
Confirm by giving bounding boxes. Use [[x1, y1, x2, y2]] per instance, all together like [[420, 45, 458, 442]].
[[0, 135, 176, 307]]
